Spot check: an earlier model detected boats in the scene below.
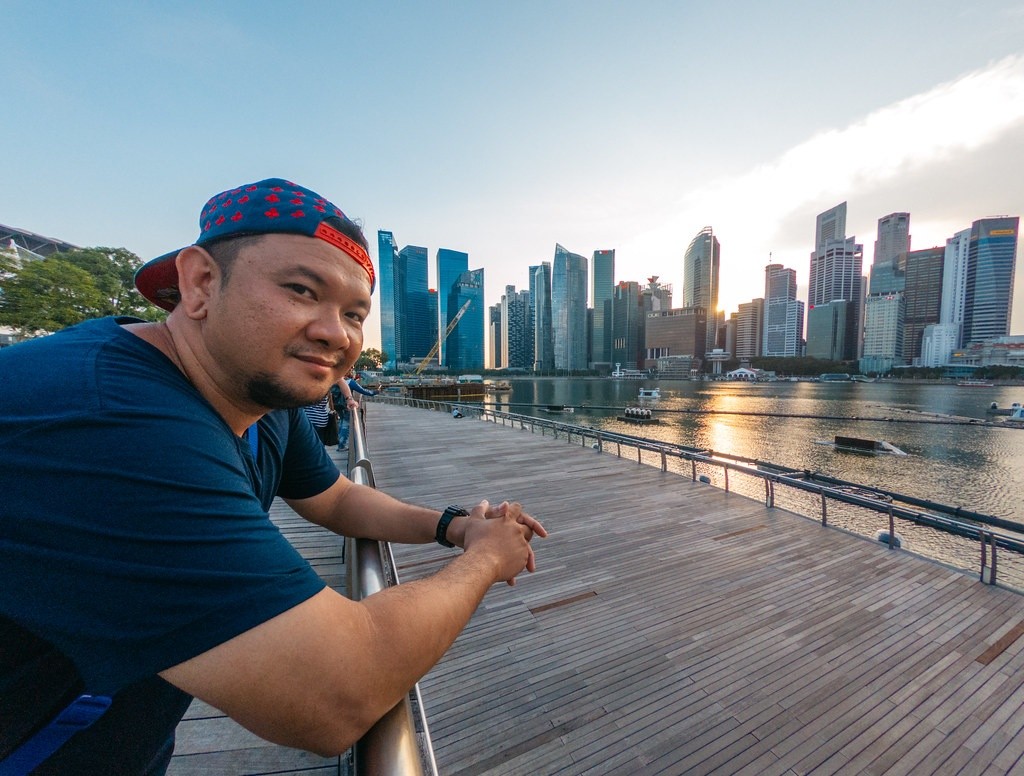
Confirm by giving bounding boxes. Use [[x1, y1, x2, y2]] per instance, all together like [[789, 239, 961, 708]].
[[483, 378, 513, 391], [538, 405, 575, 414], [823, 380, 853, 384], [832, 433, 908, 459], [987, 400, 1024, 421], [609, 362, 648, 380], [638, 387, 661, 401], [956, 380, 995, 387]]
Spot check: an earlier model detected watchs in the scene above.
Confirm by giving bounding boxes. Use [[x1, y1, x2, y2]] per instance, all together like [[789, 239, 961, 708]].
[[434, 505, 471, 548]]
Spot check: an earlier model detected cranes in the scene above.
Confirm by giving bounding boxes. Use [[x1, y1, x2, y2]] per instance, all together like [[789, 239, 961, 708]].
[[411, 294, 472, 376]]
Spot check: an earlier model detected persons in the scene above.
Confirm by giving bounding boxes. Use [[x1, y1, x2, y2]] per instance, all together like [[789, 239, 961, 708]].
[[0, 176, 548, 775], [452, 408, 466, 418], [303, 365, 382, 452]]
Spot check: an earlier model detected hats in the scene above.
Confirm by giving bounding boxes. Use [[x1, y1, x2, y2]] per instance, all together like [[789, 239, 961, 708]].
[[134, 178, 375, 313]]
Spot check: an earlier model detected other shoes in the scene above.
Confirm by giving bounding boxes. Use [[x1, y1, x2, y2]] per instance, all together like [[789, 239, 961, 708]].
[[337, 445, 349, 451]]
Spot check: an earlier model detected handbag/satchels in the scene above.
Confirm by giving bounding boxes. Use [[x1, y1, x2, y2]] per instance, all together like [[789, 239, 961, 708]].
[[325, 409, 339, 445]]
